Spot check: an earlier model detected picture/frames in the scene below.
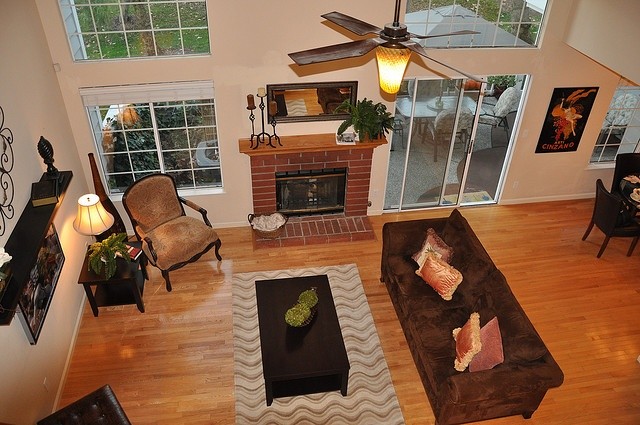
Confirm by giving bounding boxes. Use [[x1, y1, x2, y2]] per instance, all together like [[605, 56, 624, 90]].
[[17, 221, 65, 346], [266, 81, 359, 124]]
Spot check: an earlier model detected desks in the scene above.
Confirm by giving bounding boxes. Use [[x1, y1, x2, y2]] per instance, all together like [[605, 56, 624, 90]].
[[78, 240, 150, 318], [396, 96, 486, 142]]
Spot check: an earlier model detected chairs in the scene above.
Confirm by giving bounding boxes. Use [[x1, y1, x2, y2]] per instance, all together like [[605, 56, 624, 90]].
[[476, 86, 522, 143], [391, 113, 407, 149], [408, 79, 441, 135], [582, 180, 640, 260], [610, 153, 640, 196], [122, 173, 222, 292], [422, 108, 474, 162]]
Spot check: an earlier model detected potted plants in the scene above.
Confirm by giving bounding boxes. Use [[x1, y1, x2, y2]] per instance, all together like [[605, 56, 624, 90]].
[[256, 87, 266, 96], [333, 98, 395, 143], [86, 231, 132, 280]]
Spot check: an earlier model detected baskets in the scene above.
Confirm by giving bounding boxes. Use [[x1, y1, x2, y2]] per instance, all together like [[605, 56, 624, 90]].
[[248, 211, 289, 238]]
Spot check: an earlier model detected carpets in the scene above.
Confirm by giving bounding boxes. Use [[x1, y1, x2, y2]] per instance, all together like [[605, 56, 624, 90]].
[[232, 262, 404, 425]]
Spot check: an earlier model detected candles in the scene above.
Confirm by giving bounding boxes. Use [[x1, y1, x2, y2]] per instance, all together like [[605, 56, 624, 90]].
[[269, 101, 278, 118], [246, 93, 256, 109]]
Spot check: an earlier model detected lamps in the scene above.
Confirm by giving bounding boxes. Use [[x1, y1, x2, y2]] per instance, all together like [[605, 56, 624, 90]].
[[72, 193, 114, 244], [376, 45, 413, 95]]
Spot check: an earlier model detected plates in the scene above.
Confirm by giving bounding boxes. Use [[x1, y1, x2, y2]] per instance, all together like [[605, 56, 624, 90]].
[[427, 102, 451, 110], [630, 193, 640, 203]]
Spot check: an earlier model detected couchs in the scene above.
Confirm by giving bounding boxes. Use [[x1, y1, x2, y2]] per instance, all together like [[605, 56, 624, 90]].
[[36, 382, 132, 425], [380, 209, 564, 425]]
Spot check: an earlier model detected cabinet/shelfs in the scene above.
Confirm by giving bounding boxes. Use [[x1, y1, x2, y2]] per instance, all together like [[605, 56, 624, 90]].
[[0, 171, 73, 326]]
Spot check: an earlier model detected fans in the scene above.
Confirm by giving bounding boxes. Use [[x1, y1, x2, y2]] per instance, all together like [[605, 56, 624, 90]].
[[287, 0, 492, 85]]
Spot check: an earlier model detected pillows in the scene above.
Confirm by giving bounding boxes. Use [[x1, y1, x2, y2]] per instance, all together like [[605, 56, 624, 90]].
[[415, 252, 464, 301], [468, 316, 505, 371], [453, 312, 482, 372], [411, 228, 456, 268]]
